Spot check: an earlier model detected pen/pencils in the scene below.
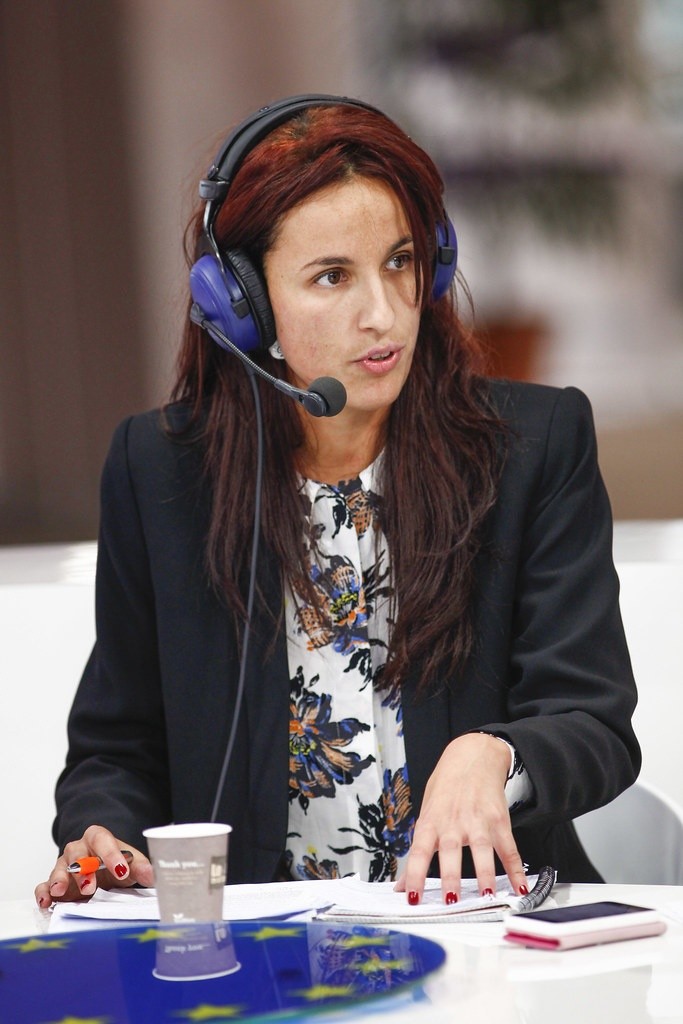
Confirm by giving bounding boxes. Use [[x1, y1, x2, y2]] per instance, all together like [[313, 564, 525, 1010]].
[[67, 850, 133, 875]]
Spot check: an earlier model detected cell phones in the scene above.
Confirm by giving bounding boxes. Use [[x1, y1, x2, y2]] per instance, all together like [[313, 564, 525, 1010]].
[[506, 901, 657, 939]]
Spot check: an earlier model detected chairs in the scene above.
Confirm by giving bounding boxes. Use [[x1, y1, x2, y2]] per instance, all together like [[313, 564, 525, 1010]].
[[572, 781, 682, 887]]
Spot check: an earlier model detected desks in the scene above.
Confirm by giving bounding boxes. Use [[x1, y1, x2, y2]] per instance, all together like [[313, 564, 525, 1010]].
[[0, 882, 683, 1023]]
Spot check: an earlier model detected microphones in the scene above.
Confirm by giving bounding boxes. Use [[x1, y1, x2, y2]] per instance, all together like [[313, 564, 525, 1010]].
[[205, 321, 347, 417]]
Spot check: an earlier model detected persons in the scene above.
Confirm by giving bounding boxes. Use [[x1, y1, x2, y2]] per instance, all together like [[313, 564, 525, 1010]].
[[32, 94, 641, 919]]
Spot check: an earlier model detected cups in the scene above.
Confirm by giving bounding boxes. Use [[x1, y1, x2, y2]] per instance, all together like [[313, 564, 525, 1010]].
[[142, 822, 232, 925]]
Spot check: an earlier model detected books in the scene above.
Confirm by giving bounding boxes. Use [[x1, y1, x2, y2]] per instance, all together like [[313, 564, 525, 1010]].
[[311, 866, 558, 925], [504, 899, 668, 952]]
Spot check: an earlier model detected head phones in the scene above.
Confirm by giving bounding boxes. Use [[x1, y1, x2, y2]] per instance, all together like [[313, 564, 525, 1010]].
[[184, 93, 457, 358]]
